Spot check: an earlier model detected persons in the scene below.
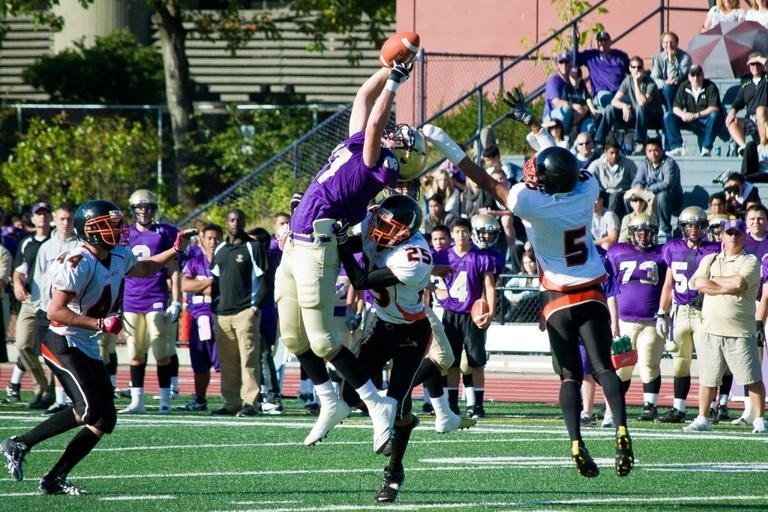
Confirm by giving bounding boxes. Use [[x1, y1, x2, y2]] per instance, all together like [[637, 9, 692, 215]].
[[279, 61, 416, 446], [623, 139, 683, 235], [727, 52, 767, 155], [30, 203, 79, 414], [562, 67, 599, 132], [440, 214, 501, 414], [570, 133, 602, 169], [746, 0, 768, 28], [602, 214, 670, 428], [504, 252, 541, 304], [0, 200, 199, 494], [484, 147, 513, 180], [291, 190, 434, 503], [421, 224, 451, 414], [11, 201, 53, 406], [117, 190, 180, 413], [701, 0, 745, 33], [432, 216, 496, 420], [13, 211, 35, 233], [485, 170, 520, 244], [298, 366, 321, 413], [6, 357, 26, 404], [421, 123, 635, 477], [0, 245, 11, 332], [591, 57, 661, 154], [714, 204, 768, 417], [682, 220, 768, 430], [722, 172, 762, 214], [753, 69, 768, 145], [334, 252, 374, 330], [525, 118, 569, 149], [578, 31, 632, 112], [247, 226, 283, 414], [651, 32, 693, 112], [421, 196, 446, 236], [210, 209, 266, 416], [180, 225, 225, 410], [585, 144, 638, 220], [706, 219, 726, 244], [427, 169, 459, 215], [664, 64, 722, 156], [618, 189, 661, 244], [97, 332, 118, 396], [657, 206, 719, 424], [269, 214, 293, 249], [591, 193, 619, 252], [705, 192, 737, 224], [543, 54, 598, 140], [754, 248, 768, 353]]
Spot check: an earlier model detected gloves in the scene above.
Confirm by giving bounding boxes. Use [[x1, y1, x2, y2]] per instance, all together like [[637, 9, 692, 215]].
[[332, 217, 350, 246], [164, 301, 183, 323], [423, 124, 466, 166], [173, 229, 199, 253], [655, 310, 670, 340], [290, 191, 304, 216], [501, 86, 535, 126], [98, 314, 123, 334], [756, 320, 765, 347], [386, 58, 414, 86]]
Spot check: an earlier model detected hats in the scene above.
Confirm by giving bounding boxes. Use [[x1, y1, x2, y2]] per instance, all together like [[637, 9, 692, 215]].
[[689, 64, 703, 74], [597, 32, 611, 40], [557, 51, 571, 62], [32, 202, 52, 214], [724, 219, 747, 236], [747, 51, 763, 64], [567, 66, 583, 79]]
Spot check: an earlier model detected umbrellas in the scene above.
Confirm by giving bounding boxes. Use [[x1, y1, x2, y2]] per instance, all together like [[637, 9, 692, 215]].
[[686, 19, 768, 78]]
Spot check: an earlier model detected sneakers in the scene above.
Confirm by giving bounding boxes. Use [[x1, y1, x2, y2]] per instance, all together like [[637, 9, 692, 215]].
[[731, 412, 754, 424], [299, 394, 319, 411], [264, 398, 283, 414], [602, 410, 615, 426], [655, 408, 686, 423], [701, 146, 711, 157], [466, 407, 473, 417], [39, 474, 88, 495], [580, 411, 596, 426], [118, 401, 145, 414], [236, 406, 258, 417], [186, 399, 207, 410], [638, 402, 658, 421], [571, 440, 600, 477], [423, 403, 434, 414], [738, 145, 746, 156], [472, 406, 486, 420], [451, 406, 461, 416], [6, 381, 21, 402], [717, 404, 726, 418], [378, 467, 405, 502], [615, 426, 634, 476], [31, 390, 56, 409], [304, 399, 350, 445], [169, 387, 179, 399], [709, 409, 718, 423], [665, 147, 686, 157], [682, 416, 711, 431], [752, 417, 768, 433], [632, 144, 643, 155], [436, 413, 477, 433], [373, 396, 398, 454], [160, 402, 172, 413], [1, 435, 28, 481], [41, 401, 67, 416], [213, 408, 235, 416]]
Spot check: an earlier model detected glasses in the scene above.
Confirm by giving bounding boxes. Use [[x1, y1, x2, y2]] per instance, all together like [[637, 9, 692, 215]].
[[629, 64, 643, 69], [578, 140, 592, 145]]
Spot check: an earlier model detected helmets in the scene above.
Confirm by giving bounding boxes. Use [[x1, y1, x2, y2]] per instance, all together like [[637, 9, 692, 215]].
[[74, 200, 130, 249], [628, 215, 657, 248], [129, 189, 158, 225], [367, 195, 423, 249], [531, 146, 578, 194], [471, 215, 502, 249], [678, 206, 709, 240], [379, 123, 428, 179]]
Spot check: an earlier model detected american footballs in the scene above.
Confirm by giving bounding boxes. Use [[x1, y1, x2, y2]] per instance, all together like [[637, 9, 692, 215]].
[[379, 32, 420, 68], [471, 300, 489, 327]]
